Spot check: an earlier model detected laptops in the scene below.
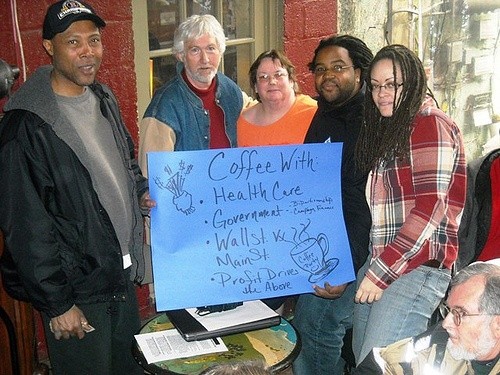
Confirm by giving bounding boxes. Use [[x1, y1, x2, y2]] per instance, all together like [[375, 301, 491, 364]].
[[165, 299, 281, 341]]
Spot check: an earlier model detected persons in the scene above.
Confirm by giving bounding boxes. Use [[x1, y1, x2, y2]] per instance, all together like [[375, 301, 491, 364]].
[[195, 358, 279, 375], [351, 263, 500, 375], [236, 49, 320, 314], [291, 33, 377, 375], [351, 43, 466, 375], [0, 0, 156, 375], [455, 128, 500, 275], [136, 14, 257, 178]]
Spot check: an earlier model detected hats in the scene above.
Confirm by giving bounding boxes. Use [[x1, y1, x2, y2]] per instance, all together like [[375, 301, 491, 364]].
[[42, 0, 106, 39]]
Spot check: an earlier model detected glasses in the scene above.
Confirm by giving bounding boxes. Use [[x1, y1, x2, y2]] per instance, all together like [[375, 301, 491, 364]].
[[367, 82, 404, 92], [256, 73, 289, 83], [439, 305, 500, 327], [315, 64, 354, 73]]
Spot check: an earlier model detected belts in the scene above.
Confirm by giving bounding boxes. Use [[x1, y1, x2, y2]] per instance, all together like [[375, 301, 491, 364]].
[[422, 258, 447, 269]]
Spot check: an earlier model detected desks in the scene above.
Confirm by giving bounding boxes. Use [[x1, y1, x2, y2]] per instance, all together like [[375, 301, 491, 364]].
[[132, 312, 301, 374]]
